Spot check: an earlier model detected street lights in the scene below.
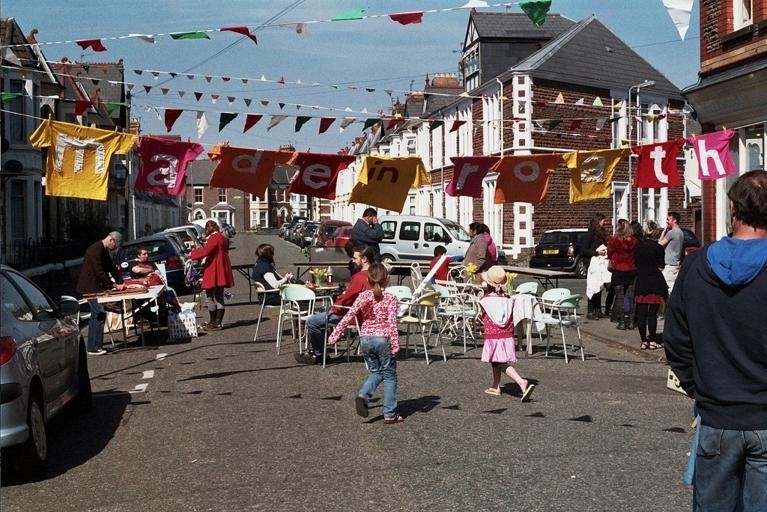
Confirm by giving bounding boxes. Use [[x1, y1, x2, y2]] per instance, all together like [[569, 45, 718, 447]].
[[629, 80, 657, 221]]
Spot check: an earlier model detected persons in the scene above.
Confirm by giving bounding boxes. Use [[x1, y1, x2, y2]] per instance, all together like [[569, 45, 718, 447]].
[[663, 170, 767, 512], [632, 220, 669, 351], [253, 244, 295, 306], [602, 221, 643, 319], [349, 208, 383, 262], [478, 265, 534, 402], [658, 212, 682, 322], [464, 222, 488, 283], [294, 242, 375, 365], [328, 263, 404, 424], [184, 220, 234, 331], [75, 232, 126, 355], [579, 213, 608, 321], [127, 248, 182, 323], [607, 219, 639, 330], [429, 245, 464, 286], [481, 224, 497, 272]]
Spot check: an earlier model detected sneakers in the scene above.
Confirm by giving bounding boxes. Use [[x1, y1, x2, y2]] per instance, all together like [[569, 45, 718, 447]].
[[99, 346, 102, 351], [88, 350, 106, 355], [294, 350, 316, 363], [310, 354, 330, 363]]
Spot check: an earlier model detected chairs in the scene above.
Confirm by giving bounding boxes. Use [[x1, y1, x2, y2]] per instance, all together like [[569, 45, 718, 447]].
[[276, 283, 317, 349], [253, 281, 296, 341], [385, 286, 412, 317], [62, 295, 117, 353], [542, 288, 575, 353], [425, 285, 477, 352], [526, 294, 585, 363], [516, 281, 538, 295], [396, 292, 447, 365], [323, 304, 372, 369]]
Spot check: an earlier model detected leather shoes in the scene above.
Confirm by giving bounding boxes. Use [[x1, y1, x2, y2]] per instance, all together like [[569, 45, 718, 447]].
[[627, 319, 634, 329], [616, 321, 625, 330]]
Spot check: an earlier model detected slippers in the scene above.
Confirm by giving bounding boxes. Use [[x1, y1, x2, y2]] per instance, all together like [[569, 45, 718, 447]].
[[521, 384, 535, 402], [484, 387, 502, 397]]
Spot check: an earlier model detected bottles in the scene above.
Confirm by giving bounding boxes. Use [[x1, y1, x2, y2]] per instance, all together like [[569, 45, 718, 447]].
[[327, 266, 334, 287]]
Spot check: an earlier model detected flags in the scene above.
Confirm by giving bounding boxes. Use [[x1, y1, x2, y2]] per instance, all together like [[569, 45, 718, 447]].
[[219, 112, 239, 132], [658, 114, 667, 120], [196, 110, 209, 139], [339, 117, 355, 135], [76, 39, 107, 52], [133, 70, 143, 75], [186, 75, 193, 80], [518, 0, 551, 29], [277, 76, 285, 84], [108, 102, 128, 119], [91, 79, 100, 86], [81, 64, 89, 73], [295, 75, 402, 118], [407, 117, 419, 128], [100, 67, 108, 73], [220, 26, 257, 47], [205, 76, 212, 82], [260, 100, 269, 106], [596, 116, 607, 131], [473, 120, 485, 135], [38, 95, 59, 114], [279, 102, 285, 109], [460, 0, 489, 8], [295, 116, 312, 132], [108, 81, 117, 86], [646, 114, 655, 123], [537, 119, 547, 128], [118, 68, 125, 74], [0, 46, 22, 68], [244, 98, 253, 107], [569, 119, 584, 133], [126, 84, 135, 90], [318, 118, 336, 135], [169, 30, 211, 41], [267, 114, 288, 131], [386, 119, 406, 131], [144, 86, 153, 94], [389, 11, 424, 24], [362, 118, 381, 131], [458, 92, 470, 97], [242, 79, 248, 86], [518, 100, 527, 107], [424, 93, 432, 100], [0, 65, 28, 78], [331, 6, 370, 21], [662, 1, 693, 41], [574, 97, 584, 107], [160, 88, 171, 96], [1, 92, 22, 111], [243, 115, 263, 133], [211, 94, 220, 102], [509, 117, 526, 123], [610, 116, 621, 124], [128, 34, 156, 46], [429, 120, 445, 132], [449, 121, 467, 133], [168, 73, 179, 80], [547, 119, 564, 131], [613, 102, 623, 108], [635, 115, 642, 122], [592, 96, 604, 107], [73, 100, 95, 117], [178, 90, 185, 98], [227, 96, 235, 103], [150, 72, 159, 80], [165, 108, 183, 132], [536, 101, 549, 115], [555, 92, 565, 104], [281, 21, 319, 35], [222, 77, 231, 82], [499, 95, 508, 100], [194, 92, 203, 101], [261, 74, 266, 81]]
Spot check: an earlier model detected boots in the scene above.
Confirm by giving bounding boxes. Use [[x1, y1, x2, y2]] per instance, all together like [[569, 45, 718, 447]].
[[200, 310, 218, 330], [203, 309, 225, 330], [587, 313, 598, 320], [595, 307, 609, 318]]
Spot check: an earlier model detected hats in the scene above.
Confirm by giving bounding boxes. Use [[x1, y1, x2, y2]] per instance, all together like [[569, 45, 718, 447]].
[[481, 265, 508, 295], [593, 242, 607, 252], [110, 231, 123, 249]]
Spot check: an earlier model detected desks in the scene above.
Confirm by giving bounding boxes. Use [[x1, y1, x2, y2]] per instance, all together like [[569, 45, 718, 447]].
[[293, 261, 350, 282], [191, 264, 256, 310], [502, 263, 577, 291]]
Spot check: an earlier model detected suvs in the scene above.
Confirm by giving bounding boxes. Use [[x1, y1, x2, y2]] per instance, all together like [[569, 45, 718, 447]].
[[294, 220, 313, 242], [377, 215, 472, 275], [311, 220, 352, 247]]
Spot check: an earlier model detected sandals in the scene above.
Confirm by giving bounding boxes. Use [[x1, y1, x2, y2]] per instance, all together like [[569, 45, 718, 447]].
[[384, 414, 404, 424], [650, 342, 662, 349], [640, 342, 649, 349], [356, 396, 369, 417]]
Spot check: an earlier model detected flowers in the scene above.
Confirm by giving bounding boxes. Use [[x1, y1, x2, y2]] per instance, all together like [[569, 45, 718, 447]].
[[505, 272, 520, 283], [465, 262, 478, 273], [309, 268, 326, 276]]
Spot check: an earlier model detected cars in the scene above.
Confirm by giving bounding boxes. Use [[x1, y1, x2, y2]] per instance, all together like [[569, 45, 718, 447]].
[[164, 228, 196, 249], [301, 224, 316, 248], [121, 232, 191, 294], [279, 223, 289, 237], [529, 228, 590, 278], [174, 223, 202, 237], [283, 224, 290, 240], [291, 223, 302, 241], [0, 264, 92, 465], [325, 226, 353, 248]]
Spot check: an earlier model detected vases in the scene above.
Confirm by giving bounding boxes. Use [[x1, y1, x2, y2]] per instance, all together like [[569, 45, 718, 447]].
[[467, 273, 474, 285], [508, 283, 514, 297], [315, 277, 321, 285]]
